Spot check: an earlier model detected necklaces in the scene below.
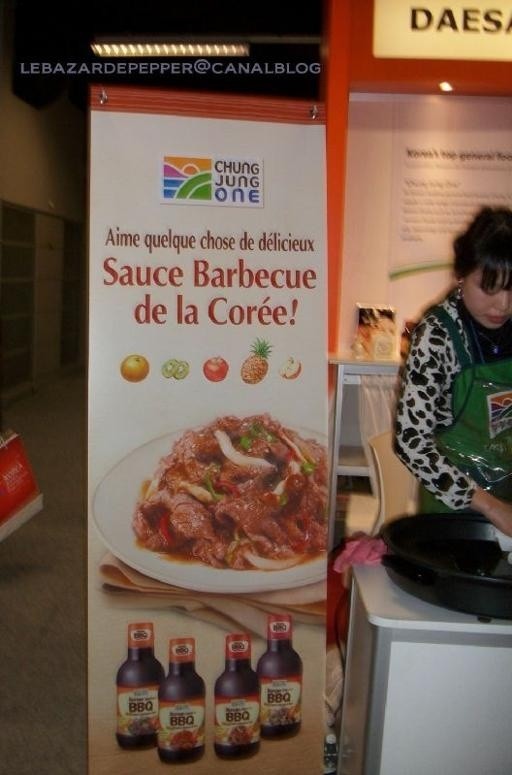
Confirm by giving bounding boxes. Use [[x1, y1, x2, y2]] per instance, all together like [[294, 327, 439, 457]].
[[475, 329, 502, 354]]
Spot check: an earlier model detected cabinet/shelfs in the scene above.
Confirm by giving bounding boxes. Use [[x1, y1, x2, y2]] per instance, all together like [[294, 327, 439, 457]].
[[326, 359, 423, 551]]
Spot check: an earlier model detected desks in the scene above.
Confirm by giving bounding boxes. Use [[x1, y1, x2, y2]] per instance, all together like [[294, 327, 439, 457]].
[[336, 568, 512, 775]]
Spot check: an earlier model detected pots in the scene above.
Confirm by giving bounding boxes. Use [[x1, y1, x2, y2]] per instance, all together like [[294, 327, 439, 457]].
[[378, 506, 510, 626]]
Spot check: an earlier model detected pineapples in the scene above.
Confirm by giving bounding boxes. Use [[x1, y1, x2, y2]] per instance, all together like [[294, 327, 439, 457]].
[[241, 336, 273, 384]]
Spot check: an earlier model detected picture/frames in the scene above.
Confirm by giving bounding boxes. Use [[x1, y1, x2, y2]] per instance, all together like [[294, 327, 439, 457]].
[[351, 300, 400, 362]]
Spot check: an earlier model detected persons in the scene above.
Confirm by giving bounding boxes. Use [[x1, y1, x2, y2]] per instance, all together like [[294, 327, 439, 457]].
[[391, 202, 511, 542]]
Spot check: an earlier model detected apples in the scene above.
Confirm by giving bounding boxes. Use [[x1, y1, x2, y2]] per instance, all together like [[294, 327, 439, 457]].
[[280, 358, 301, 379], [203, 358, 228, 383]]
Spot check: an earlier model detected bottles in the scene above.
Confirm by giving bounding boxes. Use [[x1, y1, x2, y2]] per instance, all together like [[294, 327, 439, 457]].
[[114, 617, 304, 765]]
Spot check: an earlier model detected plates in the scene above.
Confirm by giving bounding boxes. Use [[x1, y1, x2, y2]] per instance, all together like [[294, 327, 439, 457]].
[[94, 419, 328, 592]]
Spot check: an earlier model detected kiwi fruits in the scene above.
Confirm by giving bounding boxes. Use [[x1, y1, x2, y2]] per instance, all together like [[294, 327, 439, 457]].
[[161, 359, 178, 378], [173, 361, 189, 379]]
